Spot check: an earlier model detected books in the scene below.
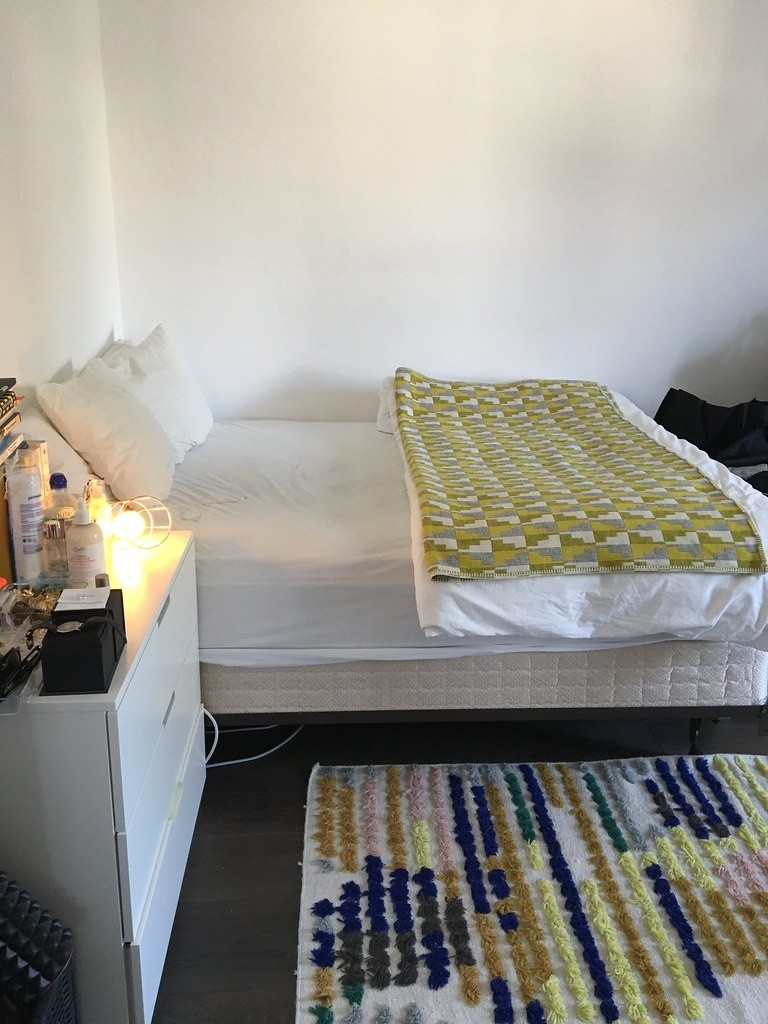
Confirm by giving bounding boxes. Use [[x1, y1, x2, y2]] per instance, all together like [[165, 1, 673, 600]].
[[25, 440, 51, 510], [0, 378, 29, 478]]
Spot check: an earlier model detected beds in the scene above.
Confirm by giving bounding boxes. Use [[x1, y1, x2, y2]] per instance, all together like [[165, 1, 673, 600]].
[[13, 339, 768, 758]]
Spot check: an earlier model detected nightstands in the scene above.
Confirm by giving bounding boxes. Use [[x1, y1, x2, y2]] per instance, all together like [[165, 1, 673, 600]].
[[0, 530, 206, 1024]]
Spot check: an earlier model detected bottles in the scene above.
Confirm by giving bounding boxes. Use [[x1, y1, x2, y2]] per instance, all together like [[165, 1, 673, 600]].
[[41, 473, 78, 578]]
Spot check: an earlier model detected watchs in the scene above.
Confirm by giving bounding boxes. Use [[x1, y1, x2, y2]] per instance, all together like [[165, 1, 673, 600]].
[[25, 616, 127, 650]]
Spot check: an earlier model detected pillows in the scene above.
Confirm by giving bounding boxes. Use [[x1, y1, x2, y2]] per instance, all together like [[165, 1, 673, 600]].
[[12, 322, 214, 505]]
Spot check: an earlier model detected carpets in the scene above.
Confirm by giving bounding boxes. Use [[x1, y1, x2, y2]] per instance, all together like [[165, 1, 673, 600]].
[[294, 753, 768, 1024]]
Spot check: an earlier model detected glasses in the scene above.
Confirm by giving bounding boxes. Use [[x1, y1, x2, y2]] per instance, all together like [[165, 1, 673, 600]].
[[0, 645, 41, 698]]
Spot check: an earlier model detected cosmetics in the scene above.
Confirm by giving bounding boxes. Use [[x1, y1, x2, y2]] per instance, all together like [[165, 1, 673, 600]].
[[5, 449, 112, 590]]
